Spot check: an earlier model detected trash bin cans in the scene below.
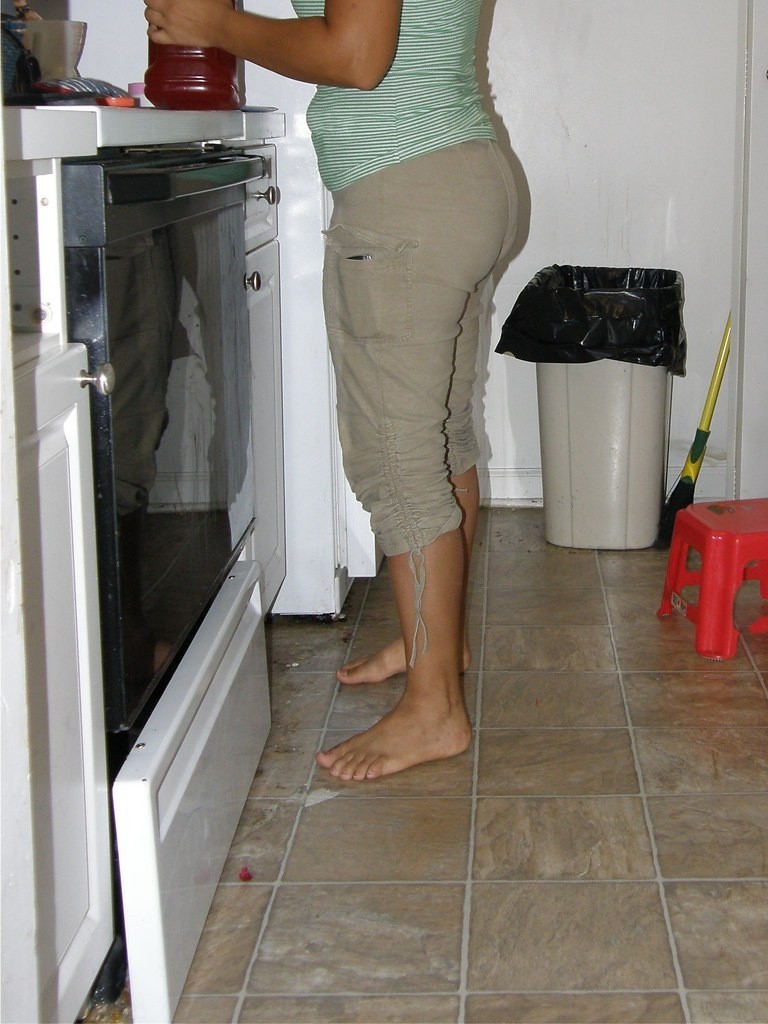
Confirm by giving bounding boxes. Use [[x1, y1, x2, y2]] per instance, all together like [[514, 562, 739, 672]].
[[525, 263, 683, 549]]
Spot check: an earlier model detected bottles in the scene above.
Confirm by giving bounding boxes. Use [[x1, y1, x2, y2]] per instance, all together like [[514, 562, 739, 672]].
[[142, 0, 241, 111]]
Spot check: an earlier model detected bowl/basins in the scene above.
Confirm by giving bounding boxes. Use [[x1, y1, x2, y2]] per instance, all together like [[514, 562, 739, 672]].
[[13, 19, 88, 82]]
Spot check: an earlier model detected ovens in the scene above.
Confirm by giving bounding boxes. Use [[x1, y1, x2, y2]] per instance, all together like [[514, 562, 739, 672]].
[[60, 142, 267, 735]]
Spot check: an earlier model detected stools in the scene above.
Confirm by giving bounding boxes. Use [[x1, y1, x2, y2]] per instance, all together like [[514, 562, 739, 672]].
[[658, 497, 768, 659]]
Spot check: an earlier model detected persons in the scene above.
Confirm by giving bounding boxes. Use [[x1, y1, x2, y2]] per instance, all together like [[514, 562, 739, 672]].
[[83, 164, 254, 688], [144, 0, 530, 781]]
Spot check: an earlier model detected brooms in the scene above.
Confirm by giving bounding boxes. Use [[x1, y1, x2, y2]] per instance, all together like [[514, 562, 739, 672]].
[[654, 308, 733, 550]]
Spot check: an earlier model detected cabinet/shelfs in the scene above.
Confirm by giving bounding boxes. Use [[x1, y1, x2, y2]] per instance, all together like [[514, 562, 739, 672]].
[[243, 143, 288, 610], [15, 351, 116, 1023]]
